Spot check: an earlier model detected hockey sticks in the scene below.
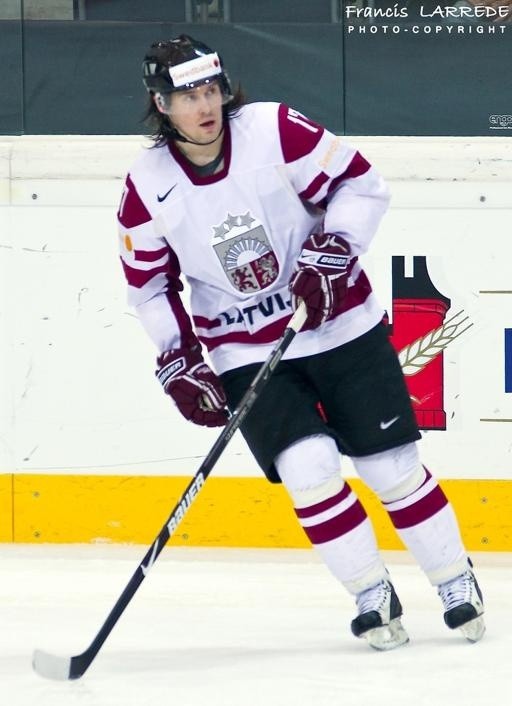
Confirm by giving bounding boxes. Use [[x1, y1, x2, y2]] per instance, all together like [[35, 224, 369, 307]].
[[32, 299, 308, 680]]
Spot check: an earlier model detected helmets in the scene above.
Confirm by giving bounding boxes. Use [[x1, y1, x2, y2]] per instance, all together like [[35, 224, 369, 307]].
[[141, 36, 235, 117]]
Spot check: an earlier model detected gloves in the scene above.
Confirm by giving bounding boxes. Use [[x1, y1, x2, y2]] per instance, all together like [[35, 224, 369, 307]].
[[151, 346, 230, 428], [293, 232, 357, 329]]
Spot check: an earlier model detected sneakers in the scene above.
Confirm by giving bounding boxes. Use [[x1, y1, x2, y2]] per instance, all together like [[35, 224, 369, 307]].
[[350, 577, 404, 635], [437, 554, 484, 629]]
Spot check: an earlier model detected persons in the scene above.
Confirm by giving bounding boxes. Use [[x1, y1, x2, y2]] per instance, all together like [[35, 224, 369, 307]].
[[112, 34, 488, 638]]
